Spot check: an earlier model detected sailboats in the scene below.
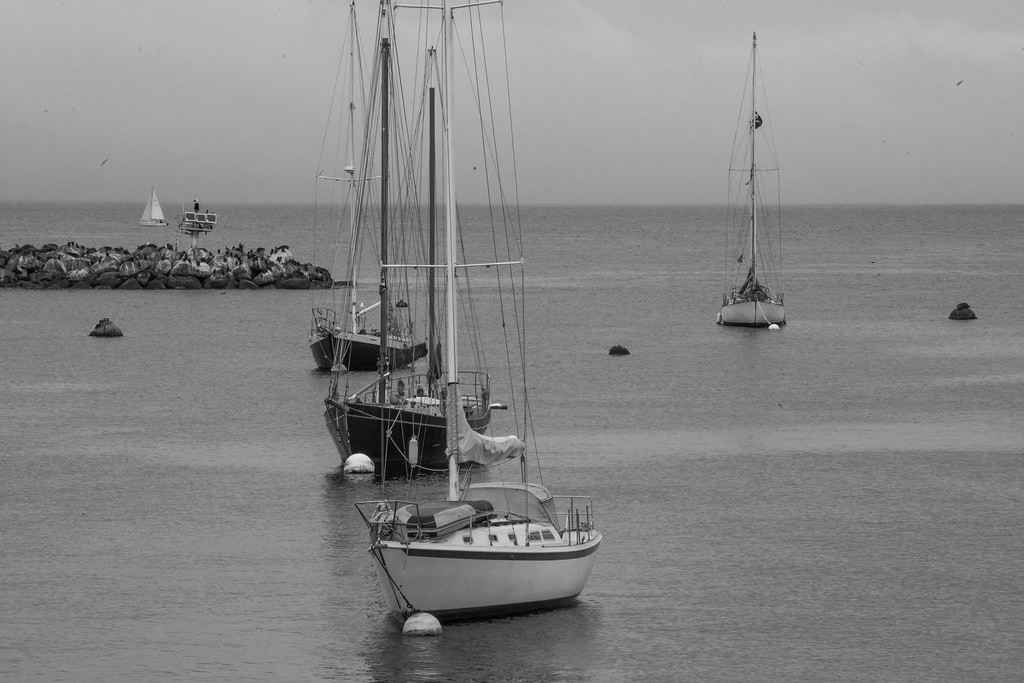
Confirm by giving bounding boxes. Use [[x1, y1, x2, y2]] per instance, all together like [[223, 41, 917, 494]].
[[138, 187, 167, 227], [322, 0, 509, 473], [716, 31, 785, 327], [308, 0, 428, 373], [354, 0, 603, 625]]
